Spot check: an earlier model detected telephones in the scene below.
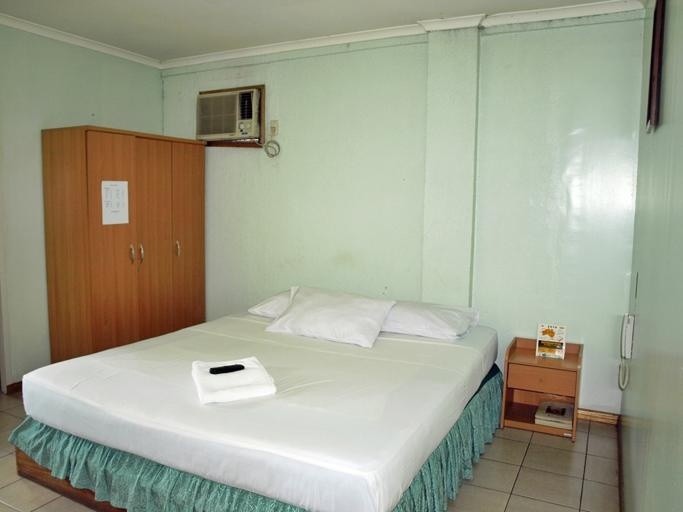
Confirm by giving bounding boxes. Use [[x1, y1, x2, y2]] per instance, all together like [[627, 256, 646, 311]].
[[621, 313, 635, 358]]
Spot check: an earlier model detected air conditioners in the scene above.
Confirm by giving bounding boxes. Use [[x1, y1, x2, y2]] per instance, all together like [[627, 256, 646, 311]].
[[194, 87, 259, 144]]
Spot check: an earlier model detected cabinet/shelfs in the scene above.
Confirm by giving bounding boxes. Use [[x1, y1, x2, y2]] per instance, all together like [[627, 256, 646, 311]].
[[39, 124, 208, 364]]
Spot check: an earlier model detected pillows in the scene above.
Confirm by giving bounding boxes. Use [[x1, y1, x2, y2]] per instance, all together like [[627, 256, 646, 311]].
[[246, 284, 481, 350]]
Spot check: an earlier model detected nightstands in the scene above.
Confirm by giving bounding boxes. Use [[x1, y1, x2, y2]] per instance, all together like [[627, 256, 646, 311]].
[[499, 336, 584, 444]]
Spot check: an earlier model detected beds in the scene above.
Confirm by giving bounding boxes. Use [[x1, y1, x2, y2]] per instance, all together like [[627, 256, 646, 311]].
[[13, 314, 505, 511]]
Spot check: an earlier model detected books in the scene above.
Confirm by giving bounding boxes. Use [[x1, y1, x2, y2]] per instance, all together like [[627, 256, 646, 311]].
[[535, 401, 573, 430]]
